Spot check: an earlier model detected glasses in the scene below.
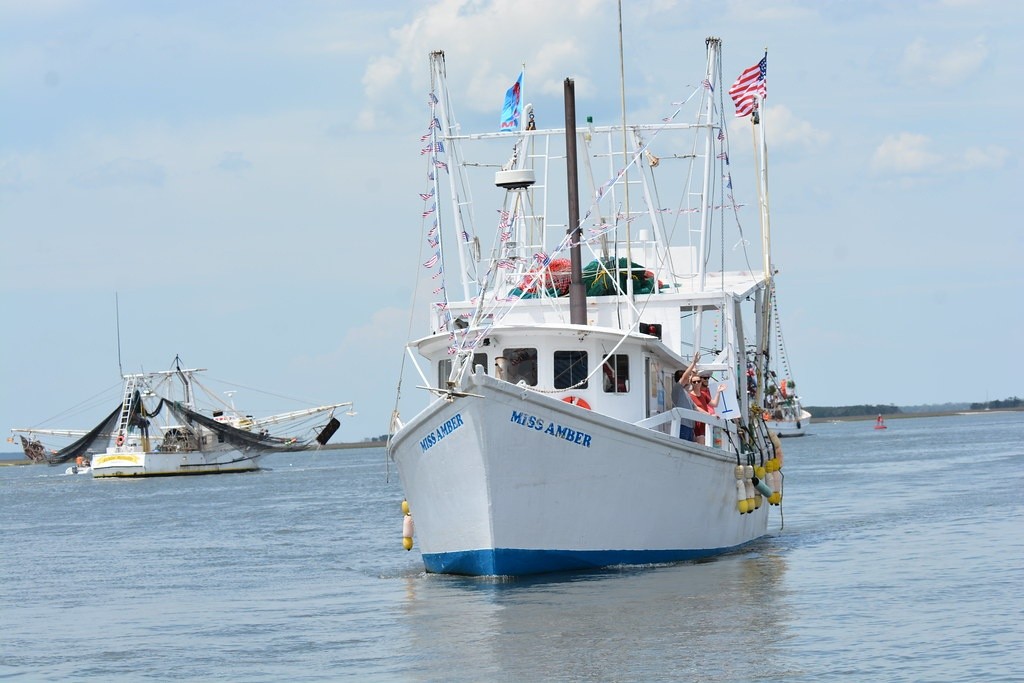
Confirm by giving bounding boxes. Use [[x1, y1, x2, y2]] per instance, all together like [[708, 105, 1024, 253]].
[[693, 380, 701, 384], [701, 377, 709, 380]]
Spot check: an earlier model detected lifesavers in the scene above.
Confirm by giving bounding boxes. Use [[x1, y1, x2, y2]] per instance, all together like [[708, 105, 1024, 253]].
[[565, 395, 591, 410], [781, 380, 787, 398], [116, 436, 125, 446]]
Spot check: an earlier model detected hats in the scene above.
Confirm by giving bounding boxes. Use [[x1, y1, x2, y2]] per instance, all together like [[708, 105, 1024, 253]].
[[697, 370, 713, 376]]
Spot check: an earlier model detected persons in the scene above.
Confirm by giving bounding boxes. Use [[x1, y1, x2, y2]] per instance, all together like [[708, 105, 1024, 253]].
[[684, 368, 727, 444], [671, 352, 701, 442]]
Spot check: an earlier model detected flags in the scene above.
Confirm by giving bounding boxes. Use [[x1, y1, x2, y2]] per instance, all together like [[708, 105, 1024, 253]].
[[420, 131, 433, 141], [585, 84, 740, 234], [727, 52, 768, 117], [447, 210, 572, 355], [701, 79, 714, 91], [498, 71, 522, 132], [429, 118, 441, 131], [717, 152, 729, 166], [428, 91, 439, 106], [418, 143, 449, 314], [718, 130, 724, 141]]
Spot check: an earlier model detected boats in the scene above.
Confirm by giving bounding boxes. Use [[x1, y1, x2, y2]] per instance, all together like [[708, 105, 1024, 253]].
[[9, 291, 357, 480], [387, 0, 812, 578], [875, 424, 887, 429]]
